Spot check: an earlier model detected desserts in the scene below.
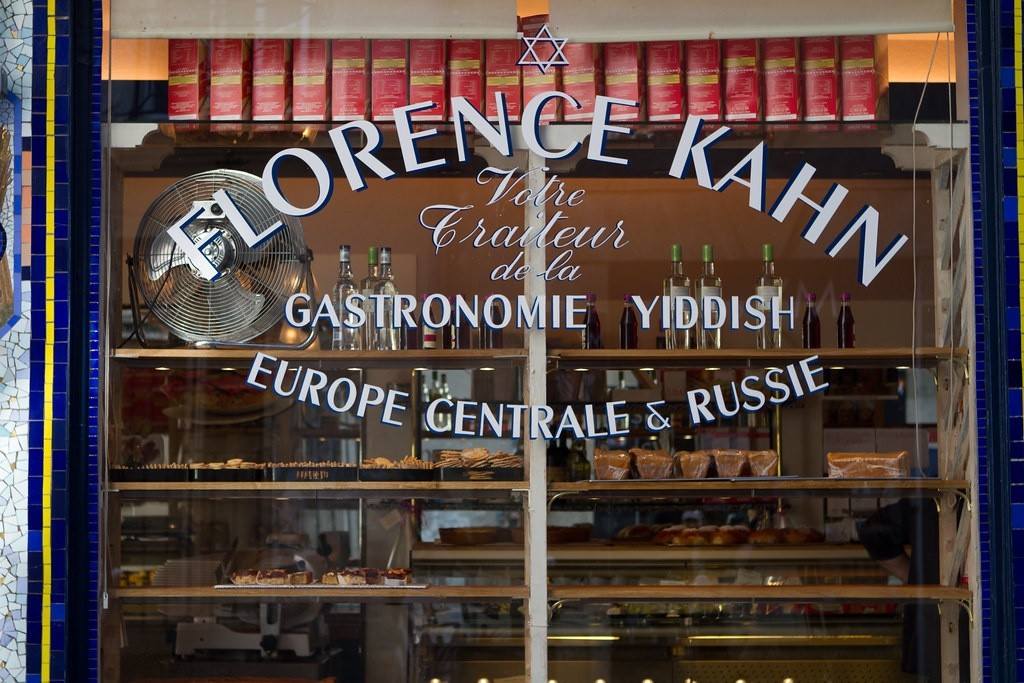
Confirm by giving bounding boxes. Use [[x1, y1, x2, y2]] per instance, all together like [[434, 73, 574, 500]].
[[230, 568, 414, 585]]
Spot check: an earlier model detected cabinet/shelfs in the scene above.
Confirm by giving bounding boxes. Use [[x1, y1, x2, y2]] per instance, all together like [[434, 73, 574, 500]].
[[108, 123, 982, 683]]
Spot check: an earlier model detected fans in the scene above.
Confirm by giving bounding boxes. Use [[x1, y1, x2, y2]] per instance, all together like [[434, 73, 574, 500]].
[[124, 168, 319, 351]]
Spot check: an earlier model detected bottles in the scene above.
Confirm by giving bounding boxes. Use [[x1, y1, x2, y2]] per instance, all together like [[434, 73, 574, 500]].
[[618, 293, 638, 351], [401, 290, 505, 351], [420, 370, 453, 432], [662, 242, 692, 351], [801, 292, 822, 350], [546, 428, 696, 481], [331, 243, 362, 351], [694, 243, 724, 351], [359, 244, 401, 351], [753, 243, 783, 350], [836, 291, 857, 349], [581, 293, 601, 350]]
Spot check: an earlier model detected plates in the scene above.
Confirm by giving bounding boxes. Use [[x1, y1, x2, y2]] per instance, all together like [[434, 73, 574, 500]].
[[163, 394, 292, 425]]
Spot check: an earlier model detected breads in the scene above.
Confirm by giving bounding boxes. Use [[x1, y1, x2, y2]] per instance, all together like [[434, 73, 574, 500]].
[[592, 448, 781, 478], [620, 523, 825, 546], [436, 524, 590, 544], [827, 450, 910, 477]]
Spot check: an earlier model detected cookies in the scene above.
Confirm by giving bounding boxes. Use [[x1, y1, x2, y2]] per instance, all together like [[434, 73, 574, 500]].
[[111, 447, 524, 479]]
